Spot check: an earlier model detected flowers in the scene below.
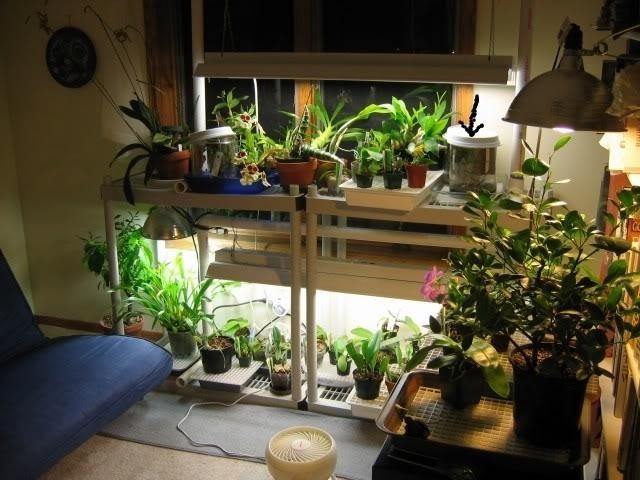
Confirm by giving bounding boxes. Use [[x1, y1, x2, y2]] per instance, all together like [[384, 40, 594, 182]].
[[381, 132, 640, 379], [220, 95, 273, 186]]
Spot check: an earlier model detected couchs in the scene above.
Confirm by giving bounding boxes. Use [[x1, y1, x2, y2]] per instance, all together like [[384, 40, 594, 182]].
[[1, 249, 176, 480]]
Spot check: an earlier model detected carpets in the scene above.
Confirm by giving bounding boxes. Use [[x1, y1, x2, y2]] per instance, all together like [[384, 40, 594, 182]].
[[98, 392, 382, 479]]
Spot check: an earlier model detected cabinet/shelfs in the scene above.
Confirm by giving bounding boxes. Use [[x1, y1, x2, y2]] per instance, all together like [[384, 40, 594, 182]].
[[97, 169, 310, 415], [299, 187, 527, 419], [583, 166, 640, 480]]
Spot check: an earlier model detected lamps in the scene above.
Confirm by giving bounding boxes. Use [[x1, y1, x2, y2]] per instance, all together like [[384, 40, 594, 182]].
[[504, 21, 628, 132], [142, 206, 198, 239]]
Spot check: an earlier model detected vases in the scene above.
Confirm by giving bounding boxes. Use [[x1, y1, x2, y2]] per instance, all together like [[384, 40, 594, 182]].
[[208, 138, 235, 182], [511, 343, 598, 451]]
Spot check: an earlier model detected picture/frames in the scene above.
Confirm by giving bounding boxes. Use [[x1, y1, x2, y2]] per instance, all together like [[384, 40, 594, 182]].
[[45, 26, 97, 88]]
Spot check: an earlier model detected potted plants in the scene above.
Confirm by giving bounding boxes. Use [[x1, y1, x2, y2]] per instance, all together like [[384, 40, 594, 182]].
[[350, 144, 381, 189], [78, 210, 163, 337], [113, 252, 242, 364], [26, 3, 200, 186], [302, 330, 331, 363], [379, 145, 405, 192], [405, 321, 517, 412], [185, 314, 247, 383], [275, 106, 345, 193], [252, 337, 271, 361], [336, 356, 352, 376], [443, 92, 506, 197], [261, 330, 300, 395], [280, 333, 299, 357], [314, 103, 397, 190], [327, 348, 342, 370], [403, 90, 463, 189], [352, 328, 388, 399], [234, 331, 257, 368], [384, 338, 415, 397]]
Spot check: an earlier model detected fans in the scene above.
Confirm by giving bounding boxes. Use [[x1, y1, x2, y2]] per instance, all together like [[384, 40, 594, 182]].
[[264, 427, 338, 480]]
